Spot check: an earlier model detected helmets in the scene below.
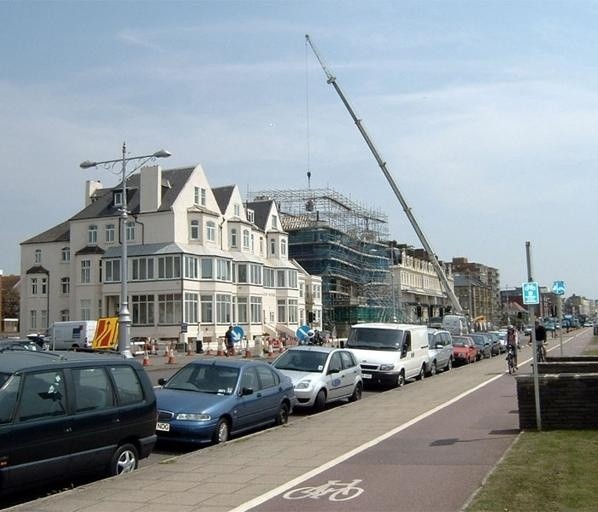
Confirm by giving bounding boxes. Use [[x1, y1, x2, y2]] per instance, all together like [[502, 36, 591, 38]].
[[507, 325, 513, 329]]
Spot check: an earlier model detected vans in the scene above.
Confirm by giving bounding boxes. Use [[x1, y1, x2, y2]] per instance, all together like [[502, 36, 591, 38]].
[[339, 323, 431, 388], [45, 321, 100, 352]]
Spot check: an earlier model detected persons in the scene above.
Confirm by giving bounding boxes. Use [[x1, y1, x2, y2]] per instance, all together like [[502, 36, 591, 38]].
[[298, 329, 323, 345], [504, 324, 519, 370], [35, 332, 43, 346], [225, 326, 234, 357], [529, 321, 547, 363]]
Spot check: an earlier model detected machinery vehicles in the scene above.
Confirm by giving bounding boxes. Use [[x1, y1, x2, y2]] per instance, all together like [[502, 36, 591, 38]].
[[305, 33, 472, 335]]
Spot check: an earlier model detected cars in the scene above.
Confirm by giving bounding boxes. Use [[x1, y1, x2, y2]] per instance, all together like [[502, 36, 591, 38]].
[[427, 325, 519, 376], [524, 313, 595, 336], [0, 333, 53, 353], [269, 346, 363, 413], [117, 337, 157, 355], [155, 357, 296, 446]]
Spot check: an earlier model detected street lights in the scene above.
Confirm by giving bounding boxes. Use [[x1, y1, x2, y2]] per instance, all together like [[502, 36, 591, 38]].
[[81, 149, 172, 348]]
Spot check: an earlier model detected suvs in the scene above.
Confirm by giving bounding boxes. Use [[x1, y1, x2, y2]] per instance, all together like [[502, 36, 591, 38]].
[[1, 350, 157, 500]]
